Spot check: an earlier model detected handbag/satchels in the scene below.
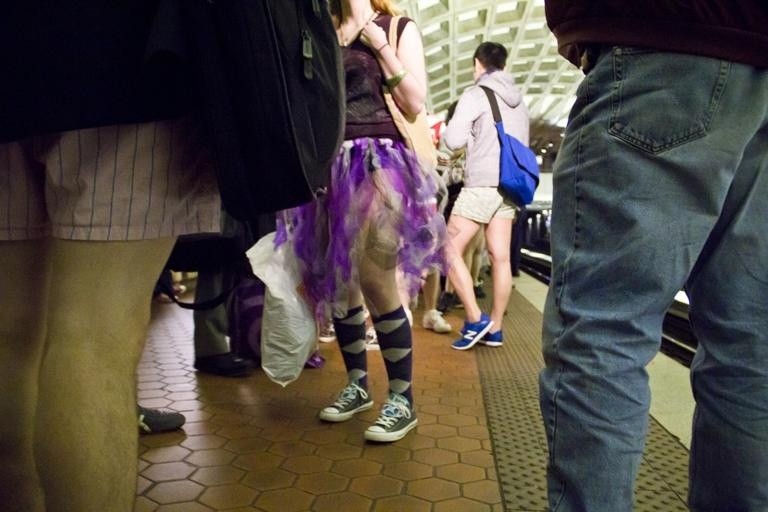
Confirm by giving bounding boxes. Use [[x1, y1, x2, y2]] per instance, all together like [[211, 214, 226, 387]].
[[184, 0, 347, 221], [486, 87, 542, 206], [386, 16, 442, 179], [164, 233, 240, 310]]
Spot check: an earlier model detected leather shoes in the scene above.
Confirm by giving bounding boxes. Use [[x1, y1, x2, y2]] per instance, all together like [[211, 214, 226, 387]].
[[194, 348, 252, 379]]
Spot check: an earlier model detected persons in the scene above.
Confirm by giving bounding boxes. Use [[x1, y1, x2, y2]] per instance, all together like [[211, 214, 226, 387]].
[[2, 0, 531, 511], [538, 0, 768, 511]]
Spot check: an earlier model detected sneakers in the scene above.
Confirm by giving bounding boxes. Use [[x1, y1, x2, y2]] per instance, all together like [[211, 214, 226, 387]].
[[486, 329, 505, 349], [140, 404, 187, 436], [439, 287, 462, 309], [319, 385, 377, 423], [453, 312, 494, 350], [476, 277, 487, 298], [363, 328, 384, 354], [366, 388, 422, 444], [419, 310, 453, 333], [319, 316, 336, 346]]
